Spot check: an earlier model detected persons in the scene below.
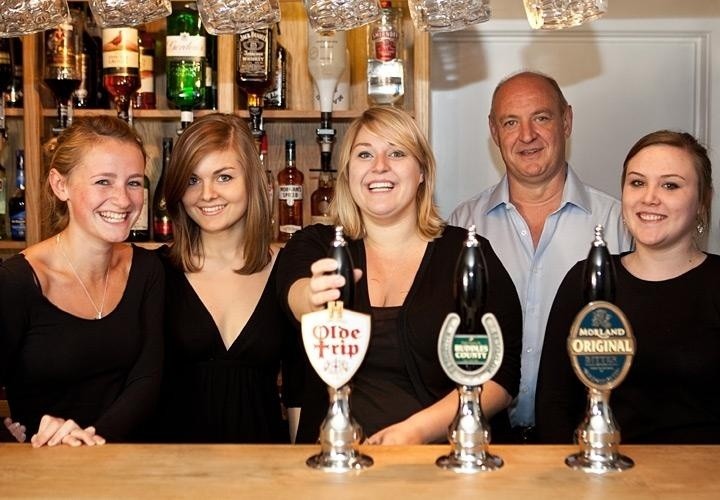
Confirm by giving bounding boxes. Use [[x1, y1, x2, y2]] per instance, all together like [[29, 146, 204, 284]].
[[0, 114, 172, 447], [151, 112, 303, 445], [277, 105, 524, 446], [446, 71, 636, 446], [534, 128, 720, 446]]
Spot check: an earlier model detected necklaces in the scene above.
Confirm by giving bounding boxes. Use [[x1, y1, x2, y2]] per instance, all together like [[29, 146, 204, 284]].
[[56, 232, 111, 320]]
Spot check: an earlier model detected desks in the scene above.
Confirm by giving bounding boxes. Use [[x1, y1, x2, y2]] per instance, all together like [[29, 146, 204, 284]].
[[4, 444, 719, 499]]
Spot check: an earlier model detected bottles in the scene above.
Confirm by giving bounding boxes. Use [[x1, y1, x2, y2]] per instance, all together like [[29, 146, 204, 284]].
[[231, 25, 277, 131], [366, 1, 405, 106], [152, 137, 173, 243], [100, 25, 141, 124], [132, 25, 157, 111], [126, 175, 149, 243], [7, 150, 27, 241], [277, 139, 304, 242], [251, 132, 276, 227], [0, 38, 14, 136], [313, 46, 351, 111], [266, 42, 287, 109], [165, 6, 209, 131], [307, 25, 346, 130], [41, 0, 84, 129], [310, 171, 337, 227]]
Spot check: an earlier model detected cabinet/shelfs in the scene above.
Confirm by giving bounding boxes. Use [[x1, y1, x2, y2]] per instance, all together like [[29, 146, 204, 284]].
[[0, 0, 431, 249]]
